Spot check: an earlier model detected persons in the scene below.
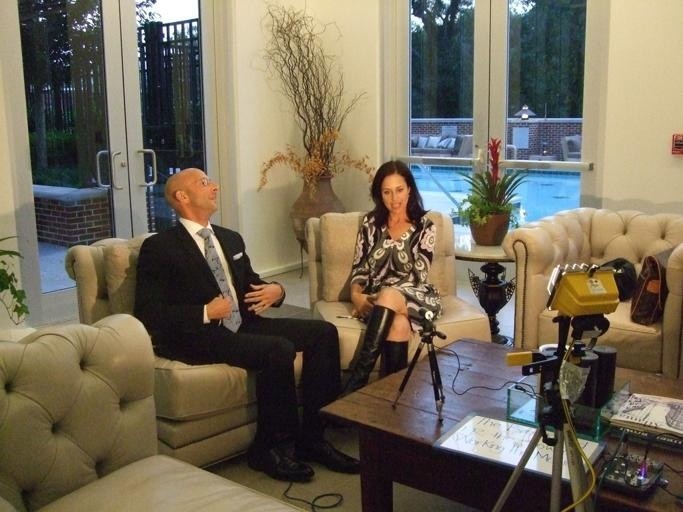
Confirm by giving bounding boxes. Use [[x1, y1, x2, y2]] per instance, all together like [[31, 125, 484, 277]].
[[339, 160, 444, 398], [133, 168, 360, 482]]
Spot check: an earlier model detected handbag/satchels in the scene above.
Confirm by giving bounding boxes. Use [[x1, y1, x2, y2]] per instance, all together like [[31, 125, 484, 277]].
[[629, 254, 668, 326], [590, 257, 637, 302]]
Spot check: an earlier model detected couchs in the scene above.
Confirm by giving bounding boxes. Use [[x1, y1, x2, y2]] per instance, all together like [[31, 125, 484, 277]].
[[0, 314, 305, 512], [411, 134, 463, 157]]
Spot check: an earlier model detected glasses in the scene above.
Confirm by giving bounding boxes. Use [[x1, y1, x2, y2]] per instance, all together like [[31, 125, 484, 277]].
[[178, 176, 212, 191]]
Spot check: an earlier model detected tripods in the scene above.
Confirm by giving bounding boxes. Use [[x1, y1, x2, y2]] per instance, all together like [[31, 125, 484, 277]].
[[490, 353, 596, 512], [391, 307, 446, 424]]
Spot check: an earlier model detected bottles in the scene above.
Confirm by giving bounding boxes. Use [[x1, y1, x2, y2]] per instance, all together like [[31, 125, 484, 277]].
[[538, 342, 566, 360], [577, 350, 598, 409], [595, 346, 617, 407]]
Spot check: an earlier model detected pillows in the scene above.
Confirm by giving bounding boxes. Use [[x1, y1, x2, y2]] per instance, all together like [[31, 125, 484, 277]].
[[417, 137, 428, 148], [427, 136, 441, 148], [411, 136, 418, 147], [447, 138, 456, 149], [439, 138, 451, 148]]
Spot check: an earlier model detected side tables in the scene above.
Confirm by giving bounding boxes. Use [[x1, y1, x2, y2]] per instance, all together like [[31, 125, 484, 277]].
[[454, 234, 516, 334]]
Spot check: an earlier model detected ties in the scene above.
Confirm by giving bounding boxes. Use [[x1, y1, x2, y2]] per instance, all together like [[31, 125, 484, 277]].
[[195, 228, 242, 334]]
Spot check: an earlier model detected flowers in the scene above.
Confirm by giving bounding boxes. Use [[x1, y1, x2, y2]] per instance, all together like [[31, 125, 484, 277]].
[[258, 1, 375, 280], [456, 138, 528, 228]]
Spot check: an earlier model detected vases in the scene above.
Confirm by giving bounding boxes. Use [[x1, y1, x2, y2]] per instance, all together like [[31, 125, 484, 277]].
[[288, 174, 347, 256], [469, 213, 510, 245]]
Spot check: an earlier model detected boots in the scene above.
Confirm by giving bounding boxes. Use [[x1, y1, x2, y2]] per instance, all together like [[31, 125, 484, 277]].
[[337, 304, 397, 400]]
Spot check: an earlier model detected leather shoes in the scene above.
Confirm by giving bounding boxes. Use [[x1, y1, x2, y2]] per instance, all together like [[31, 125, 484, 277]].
[[246, 440, 315, 480], [300, 438, 362, 474]]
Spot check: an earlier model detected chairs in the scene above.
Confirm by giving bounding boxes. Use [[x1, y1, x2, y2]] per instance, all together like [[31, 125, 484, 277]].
[[306, 212, 492, 428], [64, 233, 305, 471], [502, 206, 683, 379]]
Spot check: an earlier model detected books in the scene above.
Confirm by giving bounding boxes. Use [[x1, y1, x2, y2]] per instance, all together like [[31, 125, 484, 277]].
[[609, 391, 683, 454]]
